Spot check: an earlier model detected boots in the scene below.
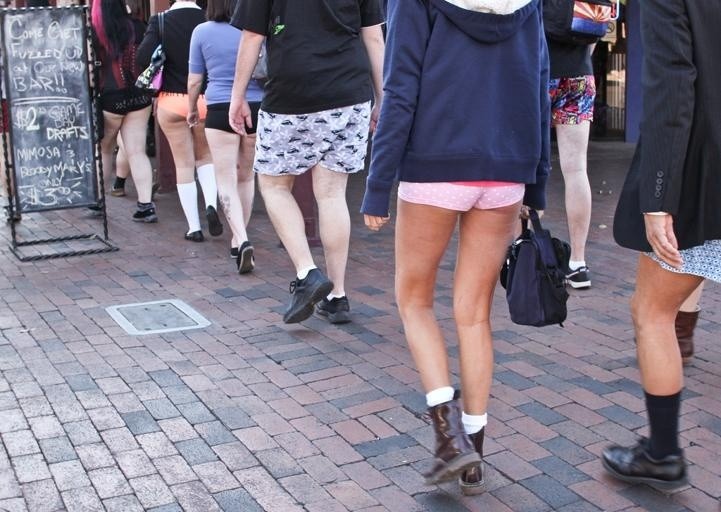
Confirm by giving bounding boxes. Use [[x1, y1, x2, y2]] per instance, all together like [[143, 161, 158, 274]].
[[460, 426, 485, 496], [422, 388, 471, 485], [674, 307, 702, 364]]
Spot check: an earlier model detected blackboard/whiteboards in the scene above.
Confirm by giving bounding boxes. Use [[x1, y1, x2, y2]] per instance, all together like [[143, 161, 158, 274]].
[[1, 7, 99, 213]]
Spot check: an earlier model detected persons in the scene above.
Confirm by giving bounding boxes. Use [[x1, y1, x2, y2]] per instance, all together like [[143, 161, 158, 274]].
[[86, 0, 159, 225], [0, 53, 22, 222], [514, 1, 619, 290], [186, 0, 266, 274], [600, 0, 721, 491], [109, 128, 161, 198], [227, 0, 388, 326], [358, 0, 552, 493], [136, 0, 225, 242], [632, 281, 705, 363]]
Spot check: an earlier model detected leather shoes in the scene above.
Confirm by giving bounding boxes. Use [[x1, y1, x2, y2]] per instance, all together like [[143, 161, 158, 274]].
[[206, 205, 223, 237], [601, 440, 688, 490], [184, 230, 204, 243]]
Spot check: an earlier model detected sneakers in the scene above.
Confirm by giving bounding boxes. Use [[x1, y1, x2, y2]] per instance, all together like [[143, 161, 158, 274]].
[[565, 265, 592, 289], [230, 241, 256, 274], [132, 201, 157, 223], [110, 185, 125, 197], [316, 295, 351, 323], [283, 268, 334, 324]]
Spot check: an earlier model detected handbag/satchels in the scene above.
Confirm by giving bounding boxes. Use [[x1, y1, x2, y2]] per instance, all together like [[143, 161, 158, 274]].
[[541, 0, 612, 46], [134, 44, 167, 97], [500, 210, 572, 327]]
